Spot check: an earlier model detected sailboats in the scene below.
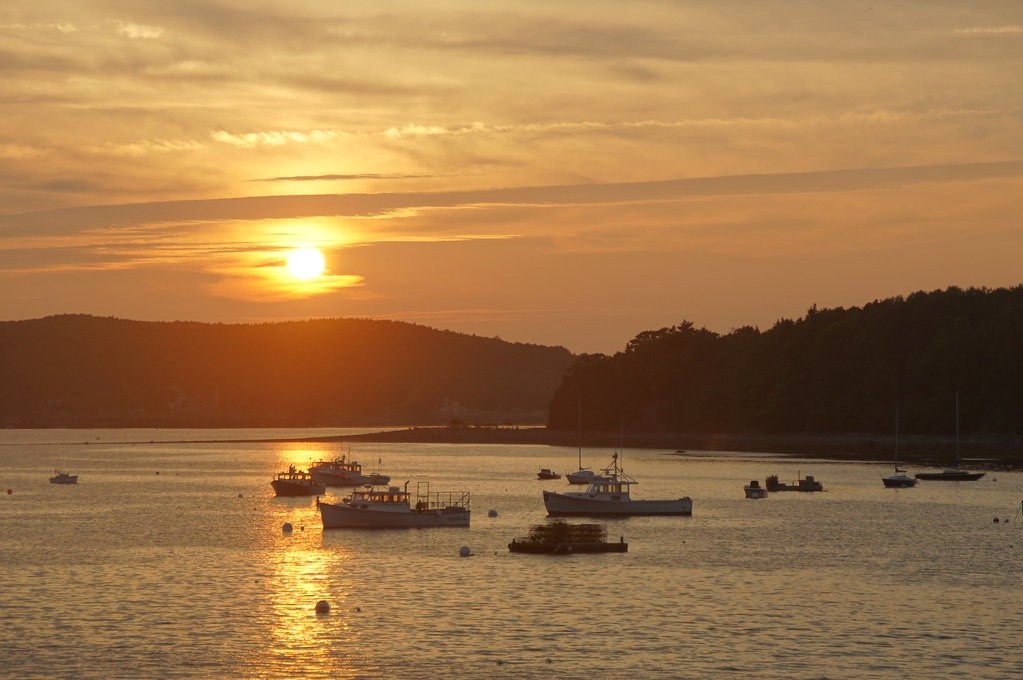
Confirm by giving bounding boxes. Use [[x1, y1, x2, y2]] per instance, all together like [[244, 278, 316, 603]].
[[881, 405, 911, 486], [914, 391, 988, 482]]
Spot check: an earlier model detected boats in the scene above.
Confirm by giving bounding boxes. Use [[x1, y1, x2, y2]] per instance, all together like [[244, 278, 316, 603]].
[[48, 469, 78, 485], [308, 441, 392, 488], [538, 468, 562, 479], [565, 397, 639, 484], [542, 449, 693, 516], [765, 467, 821, 490], [269, 465, 327, 497], [743, 479, 769, 499], [318, 479, 471, 528], [507, 517, 629, 555]]
